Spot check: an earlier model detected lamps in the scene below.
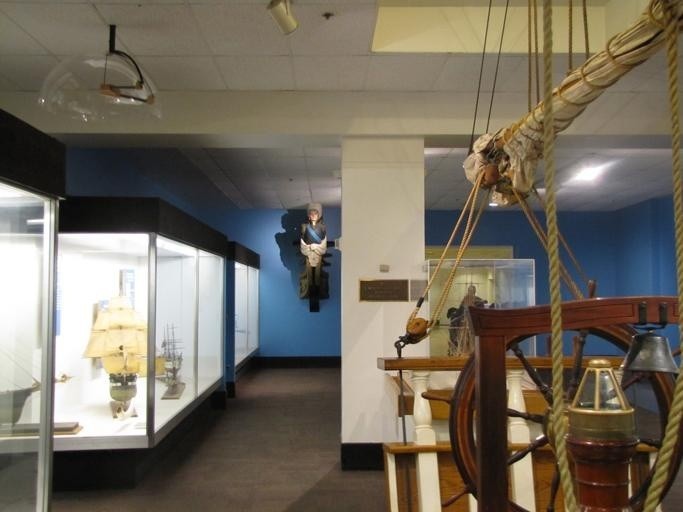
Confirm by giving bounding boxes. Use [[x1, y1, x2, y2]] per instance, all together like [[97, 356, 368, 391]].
[[268, 0, 298, 35]]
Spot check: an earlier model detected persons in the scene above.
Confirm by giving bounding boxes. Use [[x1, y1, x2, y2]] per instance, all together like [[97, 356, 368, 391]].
[[297, 202, 328, 285], [454, 284, 486, 357]]
